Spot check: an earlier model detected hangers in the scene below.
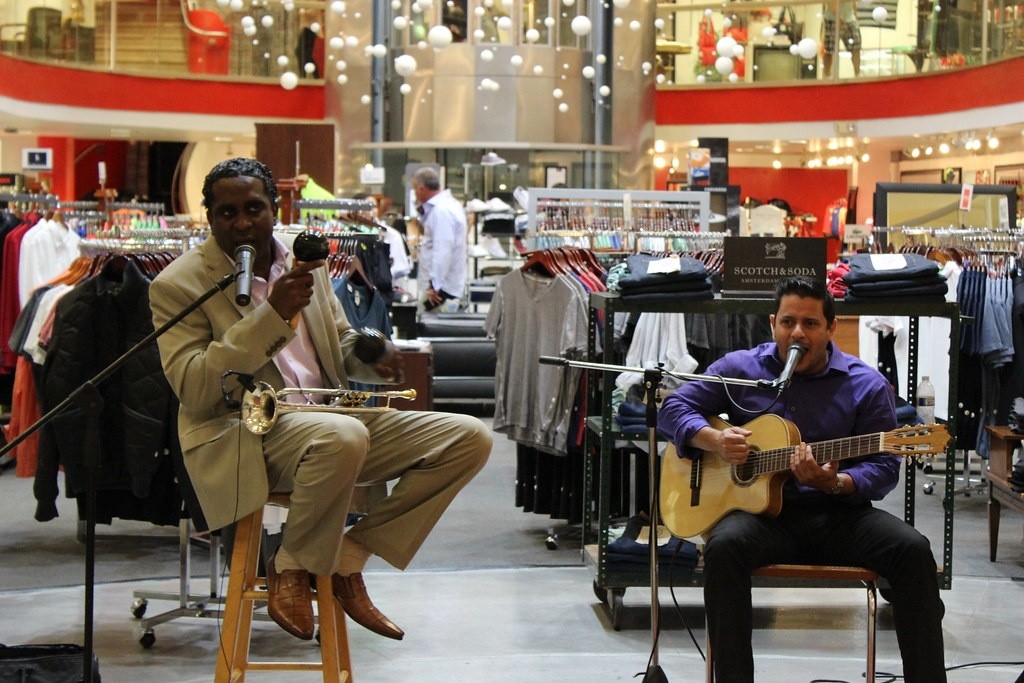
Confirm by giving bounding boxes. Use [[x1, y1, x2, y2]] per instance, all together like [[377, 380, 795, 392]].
[[647, 232, 725, 279], [8, 191, 212, 289], [866, 226, 1024, 279], [522, 229, 625, 278], [300, 198, 386, 296]]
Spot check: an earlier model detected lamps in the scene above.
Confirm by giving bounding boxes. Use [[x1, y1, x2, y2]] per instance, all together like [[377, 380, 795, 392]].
[[768, 129, 1000, 169]]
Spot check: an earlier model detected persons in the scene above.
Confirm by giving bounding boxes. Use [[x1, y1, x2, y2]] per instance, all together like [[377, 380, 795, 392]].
[[822, 0, 862, 78], [660, 280, 948, 683], [354, 195, 409, 289], [413, 168, 468, 324], [294, 7, 323, 80], [149, 158, 493, 641]]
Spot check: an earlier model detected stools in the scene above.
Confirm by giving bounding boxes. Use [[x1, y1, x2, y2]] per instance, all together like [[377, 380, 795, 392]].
[[215, 492, 353, 683], [705, 562, 878, 683]]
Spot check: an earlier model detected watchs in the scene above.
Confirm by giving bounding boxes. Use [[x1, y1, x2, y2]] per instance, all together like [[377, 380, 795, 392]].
[[824, 475, 844, 497]]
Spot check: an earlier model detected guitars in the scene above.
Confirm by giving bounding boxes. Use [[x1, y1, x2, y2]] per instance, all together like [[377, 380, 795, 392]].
[[658, 412, 955, 547]]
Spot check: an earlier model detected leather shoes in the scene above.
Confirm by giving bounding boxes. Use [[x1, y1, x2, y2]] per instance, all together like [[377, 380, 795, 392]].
[[309, 572, 404, 640], [266, 544, 314, 641]]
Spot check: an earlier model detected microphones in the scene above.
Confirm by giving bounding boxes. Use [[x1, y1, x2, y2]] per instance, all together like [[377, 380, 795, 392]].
[[234, 244, 257, 306], [237, 375, 258, 393], [778, 343, 806, 392]]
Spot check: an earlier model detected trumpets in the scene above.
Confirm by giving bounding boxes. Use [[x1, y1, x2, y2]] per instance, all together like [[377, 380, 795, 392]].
[[241, 380, 418, 435]]
